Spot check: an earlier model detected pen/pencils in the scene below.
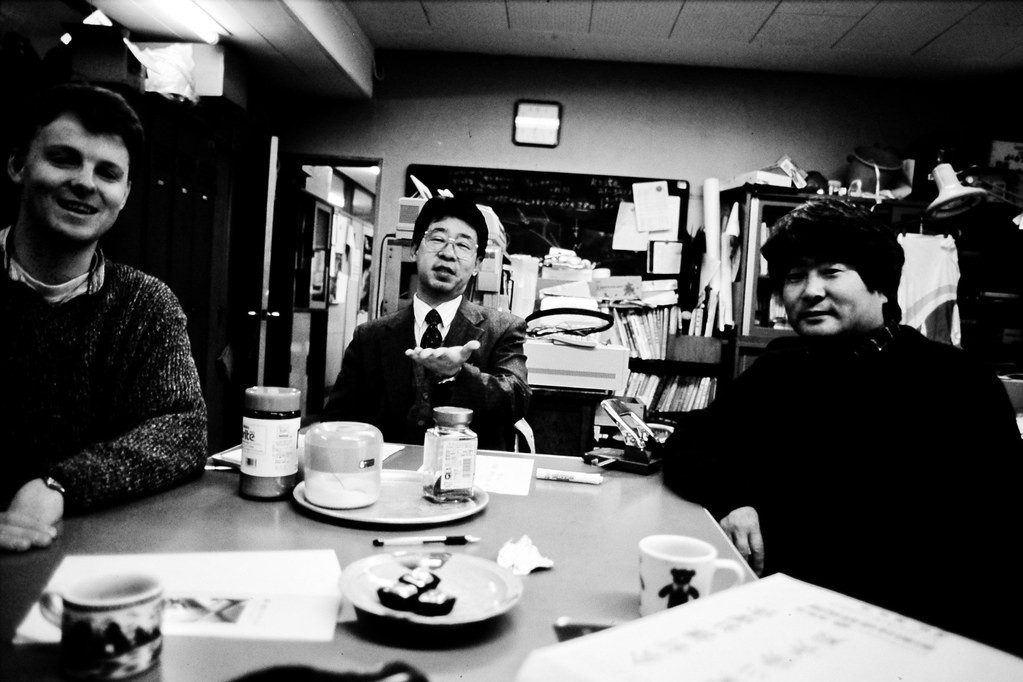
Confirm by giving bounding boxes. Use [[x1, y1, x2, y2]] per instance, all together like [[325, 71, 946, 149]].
[[370, 535, 485, 548]]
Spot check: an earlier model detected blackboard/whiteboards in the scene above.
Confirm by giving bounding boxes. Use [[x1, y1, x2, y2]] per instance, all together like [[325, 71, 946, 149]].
[[404, 162, 690, 279]]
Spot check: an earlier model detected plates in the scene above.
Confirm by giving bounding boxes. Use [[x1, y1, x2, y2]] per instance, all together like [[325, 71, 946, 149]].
[[340, 548, 523, 624], [292, 469, 489, 524]]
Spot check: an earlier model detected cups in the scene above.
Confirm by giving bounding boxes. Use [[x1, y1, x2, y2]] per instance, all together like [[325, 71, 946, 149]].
[[38, 564, 165, 681], [637, 534, 744, 618]]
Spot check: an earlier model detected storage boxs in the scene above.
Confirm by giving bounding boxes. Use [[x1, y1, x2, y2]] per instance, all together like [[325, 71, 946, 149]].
[[524, 344, 631, 389]]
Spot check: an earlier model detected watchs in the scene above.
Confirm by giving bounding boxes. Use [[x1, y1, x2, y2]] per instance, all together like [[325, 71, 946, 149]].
[[42, 475, 66, 494], [438, 367, 461, 384]]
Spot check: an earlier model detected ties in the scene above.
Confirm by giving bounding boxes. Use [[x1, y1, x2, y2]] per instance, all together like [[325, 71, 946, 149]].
[[420, 309, 442, 379]]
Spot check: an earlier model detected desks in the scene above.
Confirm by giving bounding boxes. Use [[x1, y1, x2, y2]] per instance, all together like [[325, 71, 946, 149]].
[[0, 446, 760, 682]]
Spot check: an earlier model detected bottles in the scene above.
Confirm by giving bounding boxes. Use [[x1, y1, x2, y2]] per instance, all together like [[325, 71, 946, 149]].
[[420, 406, 478, 504], [238, 386, 303, 501]]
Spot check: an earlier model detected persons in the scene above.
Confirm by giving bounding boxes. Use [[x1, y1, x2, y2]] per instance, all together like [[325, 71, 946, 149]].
[[318, 197, 531, 452], [0, 88, 208, 549], [663, 199, 1023, 657]]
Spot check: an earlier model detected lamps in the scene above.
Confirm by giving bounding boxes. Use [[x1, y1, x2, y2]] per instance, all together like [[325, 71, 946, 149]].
[[918, 162, 990, 232]]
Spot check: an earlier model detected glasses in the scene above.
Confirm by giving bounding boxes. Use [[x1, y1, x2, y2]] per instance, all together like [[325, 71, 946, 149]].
[[423, 231, 479, 261]]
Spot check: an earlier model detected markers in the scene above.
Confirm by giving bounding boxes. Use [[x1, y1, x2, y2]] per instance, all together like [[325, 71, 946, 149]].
[[536, 467, 604, 485]]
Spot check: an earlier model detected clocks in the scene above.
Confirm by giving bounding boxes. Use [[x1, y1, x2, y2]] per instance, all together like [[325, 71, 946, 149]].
[[513, 100, 562, 148]]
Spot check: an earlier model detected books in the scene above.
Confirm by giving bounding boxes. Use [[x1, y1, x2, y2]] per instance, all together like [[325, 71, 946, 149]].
[[605, 369, 717, 423], [622, 290, 718, 360]]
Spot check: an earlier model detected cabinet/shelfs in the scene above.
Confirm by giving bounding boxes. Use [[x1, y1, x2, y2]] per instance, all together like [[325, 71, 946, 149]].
[[709, 181, 926, 392]]
